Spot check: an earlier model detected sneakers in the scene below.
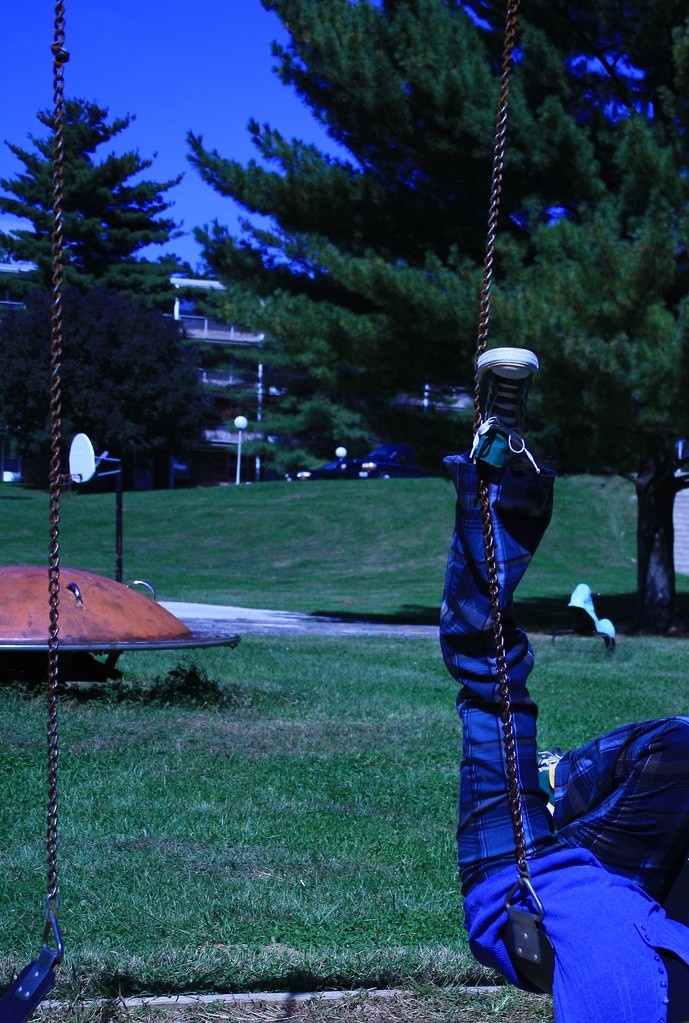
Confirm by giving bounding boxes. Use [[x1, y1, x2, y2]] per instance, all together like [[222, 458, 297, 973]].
[[538, 746, 563, 798], [469, 347, 541, 473]]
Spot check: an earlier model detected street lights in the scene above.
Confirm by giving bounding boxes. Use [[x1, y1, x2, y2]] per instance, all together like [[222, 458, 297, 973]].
[[233, 416, 249, 486]]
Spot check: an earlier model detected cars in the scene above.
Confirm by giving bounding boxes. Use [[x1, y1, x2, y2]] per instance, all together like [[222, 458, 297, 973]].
[[285, 454, 365, 479], [346, 442, 454, 479]]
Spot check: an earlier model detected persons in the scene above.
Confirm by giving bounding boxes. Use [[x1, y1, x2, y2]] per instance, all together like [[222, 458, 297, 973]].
[[441, 348, 689, 1022]]
